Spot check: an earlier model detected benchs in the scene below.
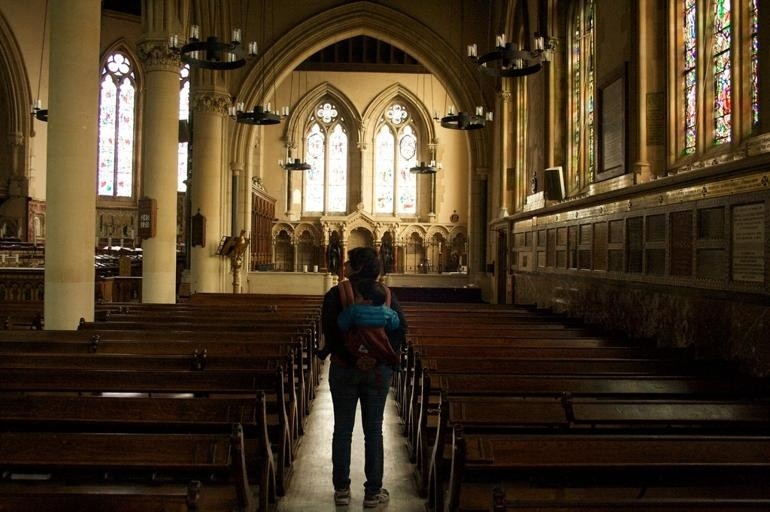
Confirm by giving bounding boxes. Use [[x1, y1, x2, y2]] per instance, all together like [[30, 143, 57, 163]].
[[0, 234, 332, 511], [386, 295, 770, 512]]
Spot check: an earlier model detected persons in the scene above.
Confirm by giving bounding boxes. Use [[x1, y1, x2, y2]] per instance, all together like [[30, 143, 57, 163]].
[[323, 246, 407, 508], [314, 279, 399, 369]]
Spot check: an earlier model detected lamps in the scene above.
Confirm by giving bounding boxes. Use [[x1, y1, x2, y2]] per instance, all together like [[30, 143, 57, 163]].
[[406, 0, 556, 175], [30, 0, 48, 122], [161, 0, 312, 171]]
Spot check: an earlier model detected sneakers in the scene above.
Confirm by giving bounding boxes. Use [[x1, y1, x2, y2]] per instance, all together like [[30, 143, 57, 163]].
[[363, 489, 389, 508], [334, 489, 350, 505]]
